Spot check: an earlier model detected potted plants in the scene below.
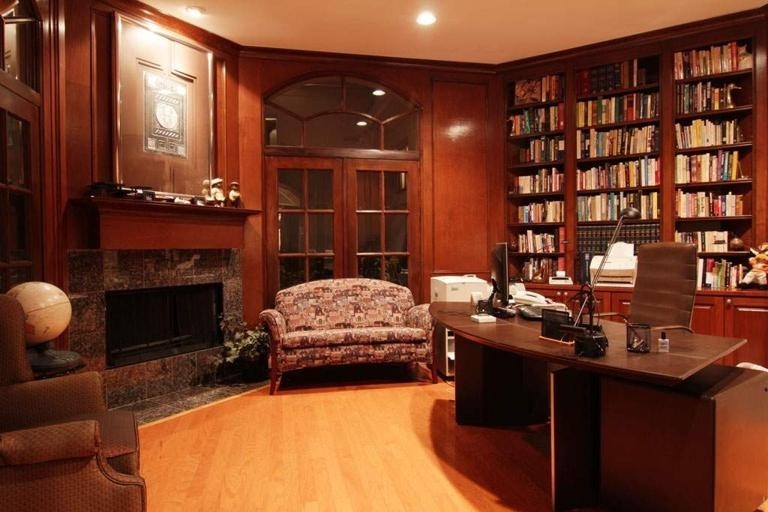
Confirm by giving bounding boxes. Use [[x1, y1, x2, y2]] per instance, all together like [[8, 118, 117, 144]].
[[201, 312, 274, 386]]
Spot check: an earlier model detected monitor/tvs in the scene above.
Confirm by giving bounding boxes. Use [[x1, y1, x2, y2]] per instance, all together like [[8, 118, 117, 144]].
[[487, 242, 516, 318]]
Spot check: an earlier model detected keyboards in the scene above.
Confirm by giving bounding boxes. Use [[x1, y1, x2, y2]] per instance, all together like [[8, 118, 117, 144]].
[[516, 306, 542, 321]]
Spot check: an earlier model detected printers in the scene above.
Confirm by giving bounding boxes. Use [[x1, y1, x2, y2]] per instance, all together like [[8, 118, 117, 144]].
[[589, 255, 637, 288]]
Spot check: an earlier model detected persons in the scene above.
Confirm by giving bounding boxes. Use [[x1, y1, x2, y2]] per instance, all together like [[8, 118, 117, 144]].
[[228, 182, 242, 208], [210, 177, 227, 208]]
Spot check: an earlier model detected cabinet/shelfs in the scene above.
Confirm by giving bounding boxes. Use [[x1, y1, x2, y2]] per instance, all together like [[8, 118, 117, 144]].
[[499, 2, 767, 370], [435, 323, 455, 379]]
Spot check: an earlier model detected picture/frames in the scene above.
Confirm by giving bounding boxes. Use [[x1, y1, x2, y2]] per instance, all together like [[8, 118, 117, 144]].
[[108, 4, 219, 206]]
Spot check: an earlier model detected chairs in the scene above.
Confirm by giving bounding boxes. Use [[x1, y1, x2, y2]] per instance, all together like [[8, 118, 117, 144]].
[[0, 294, 145, 511]]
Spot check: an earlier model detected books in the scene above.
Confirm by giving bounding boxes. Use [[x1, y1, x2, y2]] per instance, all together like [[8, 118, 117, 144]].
[[508, 74, 566, 283], [574, 57, 662, 222], [672, 41, 754, 290]]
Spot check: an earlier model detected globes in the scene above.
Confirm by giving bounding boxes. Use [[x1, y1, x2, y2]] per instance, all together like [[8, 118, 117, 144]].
[[6, 281, 86, 374]]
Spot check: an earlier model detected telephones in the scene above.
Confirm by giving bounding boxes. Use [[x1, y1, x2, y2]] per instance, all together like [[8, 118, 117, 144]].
[[513, 291, 548, 305]]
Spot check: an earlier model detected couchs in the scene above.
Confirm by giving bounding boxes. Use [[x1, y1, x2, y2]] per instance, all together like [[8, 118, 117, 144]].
[[260, 277, 434, 394]]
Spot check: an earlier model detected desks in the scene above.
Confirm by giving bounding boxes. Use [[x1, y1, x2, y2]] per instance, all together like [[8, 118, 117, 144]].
[[29, 358, 88, 378], [428, 300, 768, 512]]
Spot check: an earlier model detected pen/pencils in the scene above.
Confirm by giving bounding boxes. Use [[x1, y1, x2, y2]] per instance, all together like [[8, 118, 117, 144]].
[[618, 314, 648, 345]]
